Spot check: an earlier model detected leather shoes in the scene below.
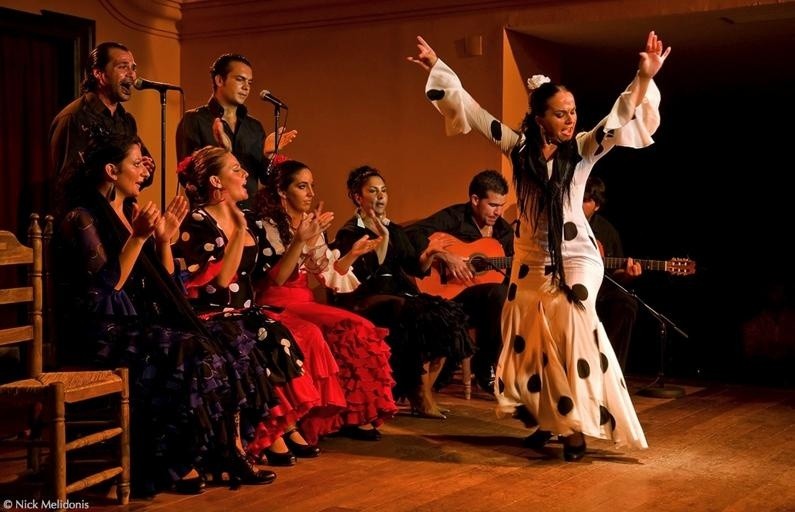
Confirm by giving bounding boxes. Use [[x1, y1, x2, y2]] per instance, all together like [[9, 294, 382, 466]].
[[470, 355, 506, 394]]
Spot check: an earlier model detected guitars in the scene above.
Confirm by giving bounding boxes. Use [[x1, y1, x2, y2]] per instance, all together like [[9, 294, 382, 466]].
[[412, 228, 517, 300], [594, 236, 698, 278]]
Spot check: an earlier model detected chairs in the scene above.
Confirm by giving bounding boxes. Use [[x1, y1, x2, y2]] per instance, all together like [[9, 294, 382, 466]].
[[0, 210, 131, 512]]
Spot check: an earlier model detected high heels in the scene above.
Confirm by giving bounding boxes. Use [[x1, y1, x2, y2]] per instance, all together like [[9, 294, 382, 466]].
[[284, 428, 322, 459], [263, 446, 298, 468], [173, 470, 207, 495], [436, 404, 452, 415], [558, 434, 590, 463], [224, 444, 277, 484], [407, 395, 447, 421], [349, 419, 380, 443], [521, 426, 554, 450]]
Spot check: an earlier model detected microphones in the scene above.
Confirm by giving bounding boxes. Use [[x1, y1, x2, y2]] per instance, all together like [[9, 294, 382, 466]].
[[259, 89, 289, 111], [131, 76, 183, 92]]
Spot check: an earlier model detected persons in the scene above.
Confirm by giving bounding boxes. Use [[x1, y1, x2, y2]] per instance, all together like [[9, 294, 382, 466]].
[[408, 171, 513, 398], [329, 168, 473, 420], [582, 179, 642, 375], [246, 154, 397, 440], [42, 41, 156, 210], [39, 136, 281, 494], [164, 145, 346, 495], [177, 54, 298, 204], [407, 31, 671, 460]]
